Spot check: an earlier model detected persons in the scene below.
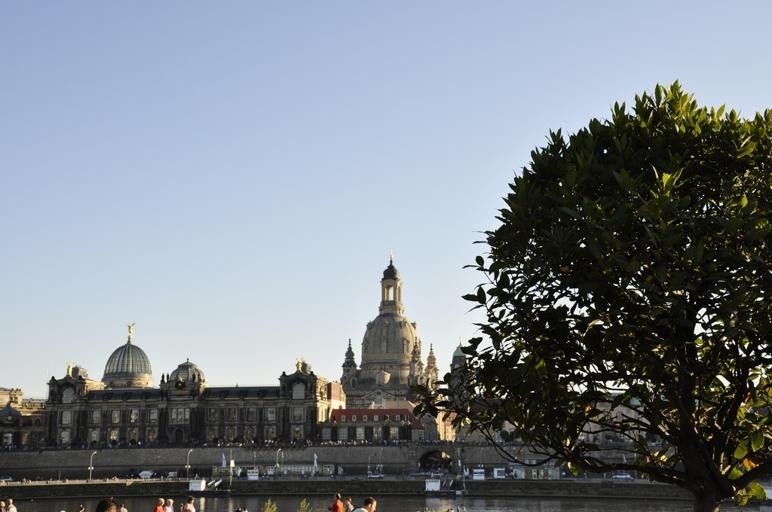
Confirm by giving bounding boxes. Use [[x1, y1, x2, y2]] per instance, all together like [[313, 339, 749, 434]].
[[0, 499, 16, 512], [237, 503, 248, 512], [504, 471, 517, 479], [425, 463, 450, 475], [327, 492, 377, 511], [384, 439, 398, 445], [1, 437, 142, 451], [217, 438, 368, 448], [301, 466, 383, 481], [153, 495, 196, 511], [416, 438, 481, 447], [236, 472, 271, 480], [95, 498, 128, 511]]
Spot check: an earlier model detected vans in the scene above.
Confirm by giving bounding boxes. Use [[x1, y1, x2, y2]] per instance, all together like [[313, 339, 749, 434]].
[[612, 473, 631, 480]]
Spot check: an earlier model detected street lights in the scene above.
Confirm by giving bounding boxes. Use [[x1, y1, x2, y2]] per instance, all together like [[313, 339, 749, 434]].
[[187, 448, 193, 480], [275, 447, 282, 478], [479, 447, 484, 480], [379, 447, 384, 480], [90, 450, 97, 482]]
